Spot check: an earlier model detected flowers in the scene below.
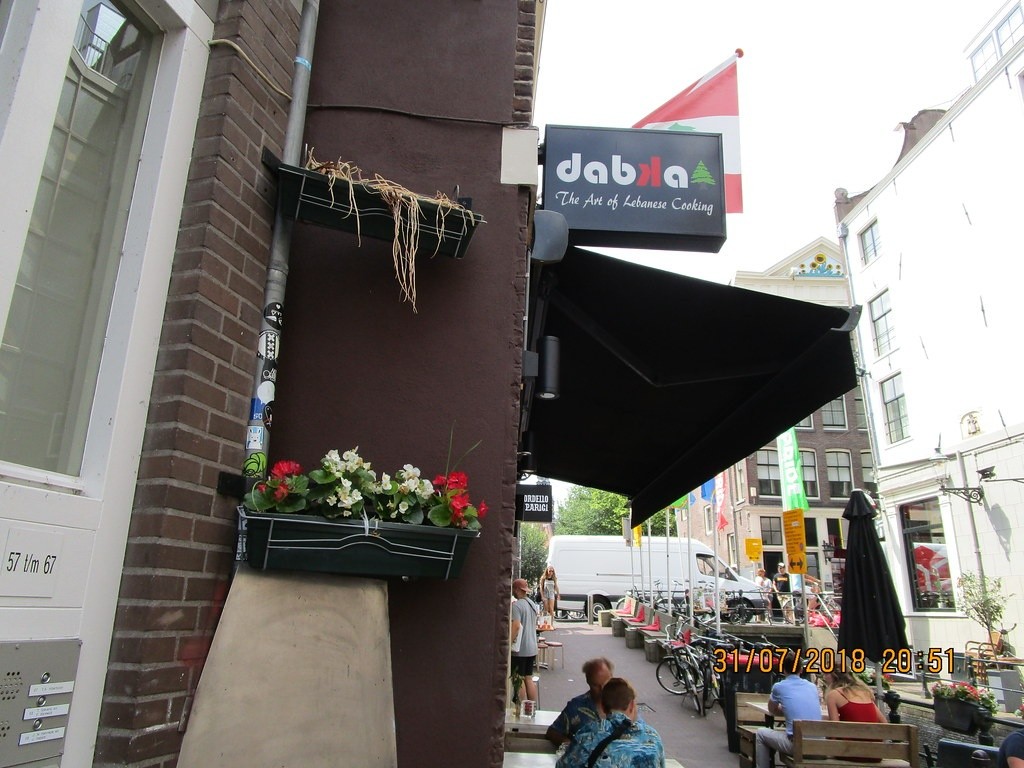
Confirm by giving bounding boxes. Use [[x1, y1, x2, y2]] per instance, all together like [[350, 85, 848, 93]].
[[856, 670, 894, 690], [930, 680, 1000, 714], [244, 419, 489, 537], [1014, 703, 1024, 719]]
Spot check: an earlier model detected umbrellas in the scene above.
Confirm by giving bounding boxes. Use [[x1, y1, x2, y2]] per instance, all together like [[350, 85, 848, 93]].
[[835, 489, 910, 712]]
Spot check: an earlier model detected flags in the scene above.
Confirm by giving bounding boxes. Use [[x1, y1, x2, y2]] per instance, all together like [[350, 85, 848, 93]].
[[632, 477, 717, 546], [631, 57, 743, 213]]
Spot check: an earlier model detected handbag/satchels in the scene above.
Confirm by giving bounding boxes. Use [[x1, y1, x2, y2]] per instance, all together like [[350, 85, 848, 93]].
[[510, 623, 524, 653], [535, 582, 544, 602]]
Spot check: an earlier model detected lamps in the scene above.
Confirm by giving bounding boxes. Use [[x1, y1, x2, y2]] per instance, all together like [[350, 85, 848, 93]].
[[822, 540, 835, 565], [523, 335, 560, 400], [930, 445, 984, 507], [976, 456, 1024, 484], [517, 431, 537, 473]]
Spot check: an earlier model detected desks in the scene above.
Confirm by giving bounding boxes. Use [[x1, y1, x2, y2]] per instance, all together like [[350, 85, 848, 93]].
[[744, 701, 840, 768], [507, 708, 562, 740], [503, 752, 685, 768], [533, 624, 555, 669]]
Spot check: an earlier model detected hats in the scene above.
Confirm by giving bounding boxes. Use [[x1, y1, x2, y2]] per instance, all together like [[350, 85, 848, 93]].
[[777, 562, 785, 568], [513, 579, 532, 593]]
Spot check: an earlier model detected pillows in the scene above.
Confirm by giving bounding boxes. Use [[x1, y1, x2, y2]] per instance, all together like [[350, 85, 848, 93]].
[[616, 598, 690, 646]]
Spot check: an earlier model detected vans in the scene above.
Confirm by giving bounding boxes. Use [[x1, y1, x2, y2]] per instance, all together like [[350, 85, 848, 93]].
[[547, 535, 770, 622]]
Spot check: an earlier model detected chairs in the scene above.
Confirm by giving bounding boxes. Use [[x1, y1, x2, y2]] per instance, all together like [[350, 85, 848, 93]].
[[504, 736, 558, 754], [964, 630, 1007, 685]]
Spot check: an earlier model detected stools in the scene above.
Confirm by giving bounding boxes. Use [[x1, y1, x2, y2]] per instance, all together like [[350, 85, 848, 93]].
[[535, 637, 563, 671], [532, 676, 541, 710]]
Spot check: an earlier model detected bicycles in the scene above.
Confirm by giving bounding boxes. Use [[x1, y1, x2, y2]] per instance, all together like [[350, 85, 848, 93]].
[[553, 608, 585, 620], [614, 579, 841, 717]]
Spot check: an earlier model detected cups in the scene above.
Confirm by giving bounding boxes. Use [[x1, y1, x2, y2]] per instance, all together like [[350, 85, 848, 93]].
[[521, 699, 536, 718]]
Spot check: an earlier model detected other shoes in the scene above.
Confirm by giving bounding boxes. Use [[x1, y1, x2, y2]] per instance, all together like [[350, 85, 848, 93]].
[[783, 620, 788, 624]]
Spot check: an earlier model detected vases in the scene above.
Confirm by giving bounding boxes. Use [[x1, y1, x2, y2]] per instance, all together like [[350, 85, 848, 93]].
[[276, 163, 481, 259], [237, 503, 479, 581], [932, 693, 984, 737]]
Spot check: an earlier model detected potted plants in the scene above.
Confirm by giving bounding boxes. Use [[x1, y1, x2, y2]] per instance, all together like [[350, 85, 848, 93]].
[[953, 570, 1023, 713], [930, 568, 947, 609]]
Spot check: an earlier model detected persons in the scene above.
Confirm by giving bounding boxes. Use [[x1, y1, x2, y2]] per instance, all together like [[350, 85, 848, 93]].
[[998, 727, 1024, 768], [756, 656, 824, 768], [555, 678, 666, 768], [511, 579, 540, 700], [546, 658, 614, 747], [540, 567, 560, 629], [773, 563, 793, 624], [825, 664, 887, 763], [755, 570, 774, 622]]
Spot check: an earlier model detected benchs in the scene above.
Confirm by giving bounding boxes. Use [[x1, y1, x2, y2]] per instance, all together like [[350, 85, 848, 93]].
[[656, 620, 700, 649], [735, 693, 771, 768], [780, 719, 920, 768], [621, 602, 654, 628], [609, 594, 638, 619], [637, 610, 675, 639]]
